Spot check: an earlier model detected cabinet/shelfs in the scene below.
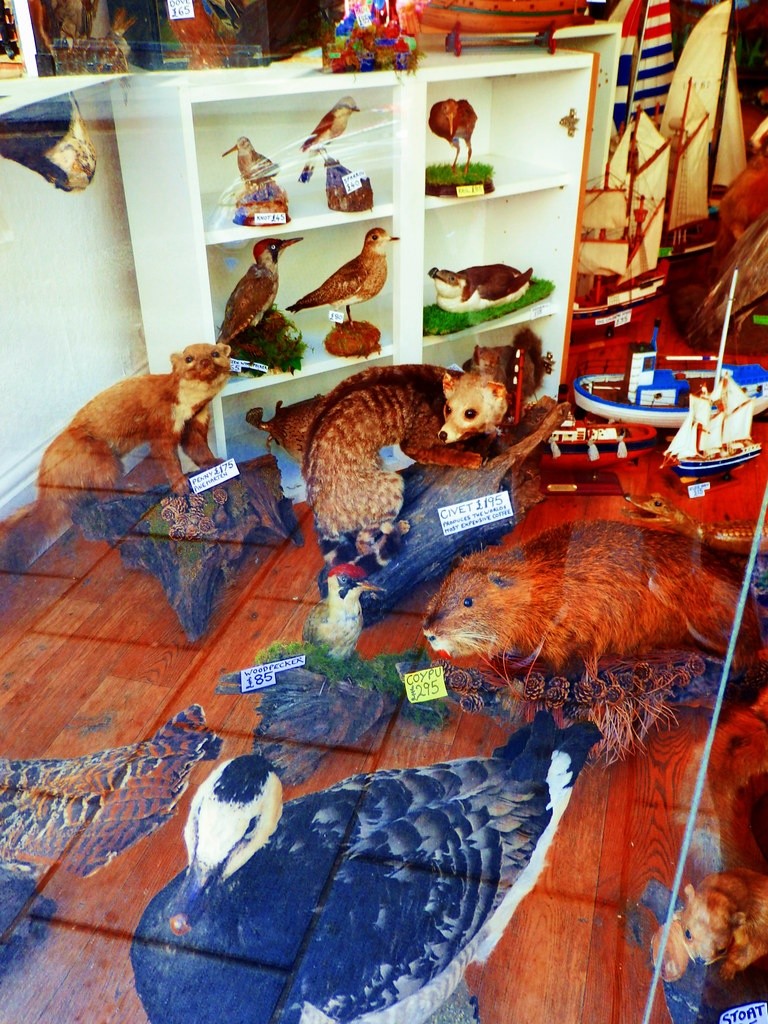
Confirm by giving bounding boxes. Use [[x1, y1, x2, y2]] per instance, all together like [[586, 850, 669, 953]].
[[106, 41, 602, 508]]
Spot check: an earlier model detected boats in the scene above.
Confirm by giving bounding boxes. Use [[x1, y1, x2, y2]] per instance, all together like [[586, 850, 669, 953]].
[[535, 421, 657, 474], [416, 0, 599, 35], [572, 268, 768, 431]]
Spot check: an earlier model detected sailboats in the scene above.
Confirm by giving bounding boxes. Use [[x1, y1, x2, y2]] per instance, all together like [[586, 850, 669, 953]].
[[566, 0, 750, 339], [660, 370, 764, 479]]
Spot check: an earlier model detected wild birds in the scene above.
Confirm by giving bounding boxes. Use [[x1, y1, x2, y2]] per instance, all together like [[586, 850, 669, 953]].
[[429, 99, 478, 178], [215, 236, 307, 345], [300, 562, 379, 664], [222, 94, 361, 202], [129, 710, 605, 1023], [284, 225, 403, 328]]
